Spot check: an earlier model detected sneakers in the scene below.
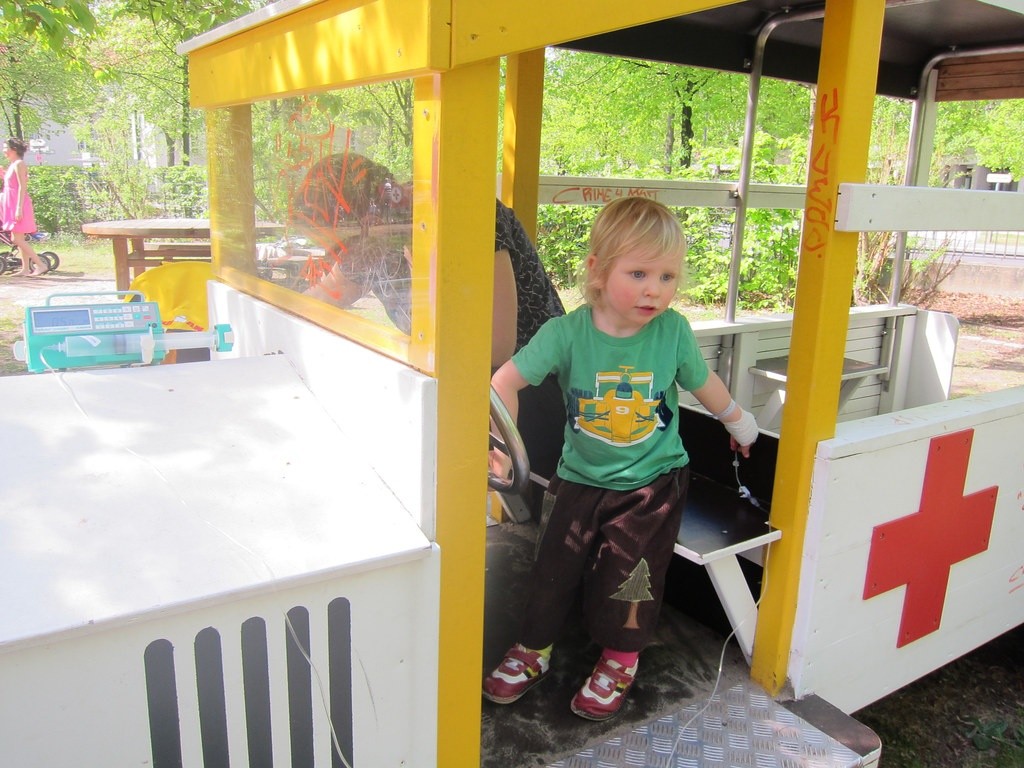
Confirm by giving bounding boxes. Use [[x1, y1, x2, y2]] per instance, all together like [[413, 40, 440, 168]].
[[571, 655, 639, 721], [482, 641, 554, 704]]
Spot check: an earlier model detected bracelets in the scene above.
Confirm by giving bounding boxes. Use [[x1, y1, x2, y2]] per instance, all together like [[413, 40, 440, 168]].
[[713, 398, 736, 420]]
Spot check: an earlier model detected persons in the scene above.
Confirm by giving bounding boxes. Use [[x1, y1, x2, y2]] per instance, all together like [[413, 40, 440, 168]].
[[480, 194, 758, 719], [1, 137, 47, 277], [294, 148, 569, 506]]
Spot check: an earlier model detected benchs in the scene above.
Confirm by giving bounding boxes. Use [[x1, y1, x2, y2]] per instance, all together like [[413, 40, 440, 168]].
[[528, 403, 783, 669]]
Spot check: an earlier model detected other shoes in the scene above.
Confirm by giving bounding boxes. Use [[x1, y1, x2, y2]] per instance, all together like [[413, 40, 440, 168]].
[[10, 269, 29, 277], [29, 264, 49, 276]]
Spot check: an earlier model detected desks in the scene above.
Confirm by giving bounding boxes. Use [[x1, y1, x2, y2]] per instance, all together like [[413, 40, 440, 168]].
[[748, 352, 888, 432], [82, 218, 288, 299]]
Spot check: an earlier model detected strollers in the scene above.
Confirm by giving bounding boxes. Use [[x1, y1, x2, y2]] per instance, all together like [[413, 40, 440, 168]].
[[0, 193, 60, 276]]
